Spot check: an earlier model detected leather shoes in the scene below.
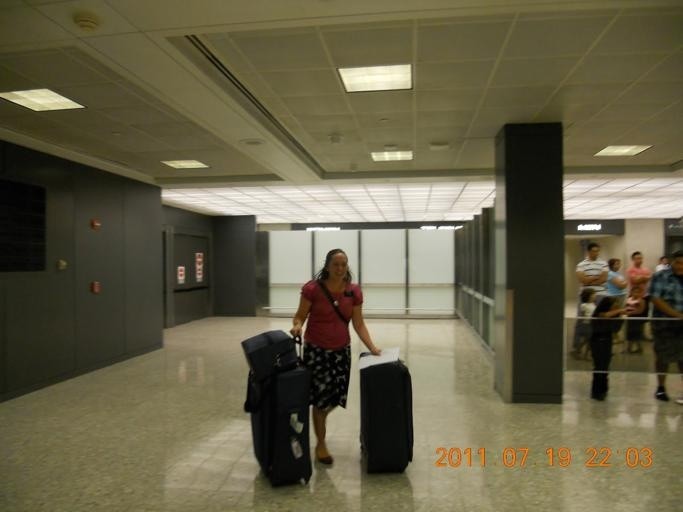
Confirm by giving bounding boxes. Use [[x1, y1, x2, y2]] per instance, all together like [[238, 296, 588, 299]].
[[319, 455, 333, 464]]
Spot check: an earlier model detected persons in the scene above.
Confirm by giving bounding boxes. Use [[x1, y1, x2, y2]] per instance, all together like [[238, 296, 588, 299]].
[[572, 241, 683, 406], [289, 248, 383, 465]]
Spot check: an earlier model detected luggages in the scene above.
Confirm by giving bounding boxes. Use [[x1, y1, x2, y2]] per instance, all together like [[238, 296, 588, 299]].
[[360, 352, 413, 474], [252, 334, 312, 486]]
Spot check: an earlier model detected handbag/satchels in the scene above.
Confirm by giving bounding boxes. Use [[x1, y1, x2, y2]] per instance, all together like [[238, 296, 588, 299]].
[[241, 330, 309, 410]]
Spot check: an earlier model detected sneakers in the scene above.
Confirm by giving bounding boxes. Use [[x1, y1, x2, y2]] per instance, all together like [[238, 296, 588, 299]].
[[654, 390, 668, 401]]
